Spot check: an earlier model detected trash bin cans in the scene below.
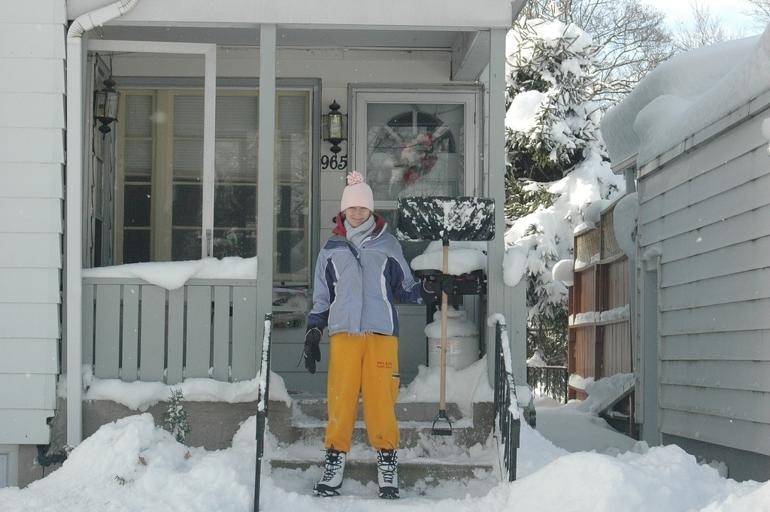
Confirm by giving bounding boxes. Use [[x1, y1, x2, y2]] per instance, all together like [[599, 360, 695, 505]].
[[423, 308, 481, 373]]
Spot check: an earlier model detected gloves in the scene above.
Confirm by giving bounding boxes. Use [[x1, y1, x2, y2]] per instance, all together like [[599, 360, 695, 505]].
[[420, 278, 457, 304], [303, 325, 324, 374]]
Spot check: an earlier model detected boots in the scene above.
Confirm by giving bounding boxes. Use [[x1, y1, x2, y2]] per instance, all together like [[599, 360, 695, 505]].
[[376, 448, 400, 499], [313, 449, 346, 497]]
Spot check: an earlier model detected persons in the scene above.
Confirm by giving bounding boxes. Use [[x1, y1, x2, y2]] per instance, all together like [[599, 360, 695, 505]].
[[300, 170, 455, 500]]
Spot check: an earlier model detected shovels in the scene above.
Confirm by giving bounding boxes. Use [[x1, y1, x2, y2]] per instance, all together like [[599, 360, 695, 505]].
[[396, 197, 495, 435]]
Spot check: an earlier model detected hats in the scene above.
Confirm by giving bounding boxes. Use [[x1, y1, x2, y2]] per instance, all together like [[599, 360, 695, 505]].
[[341, 174, 374, 214]]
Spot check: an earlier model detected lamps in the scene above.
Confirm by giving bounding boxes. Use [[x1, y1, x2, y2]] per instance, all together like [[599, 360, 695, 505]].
[[319, 98, 350, 155], [91, 76, 121, 136]]
